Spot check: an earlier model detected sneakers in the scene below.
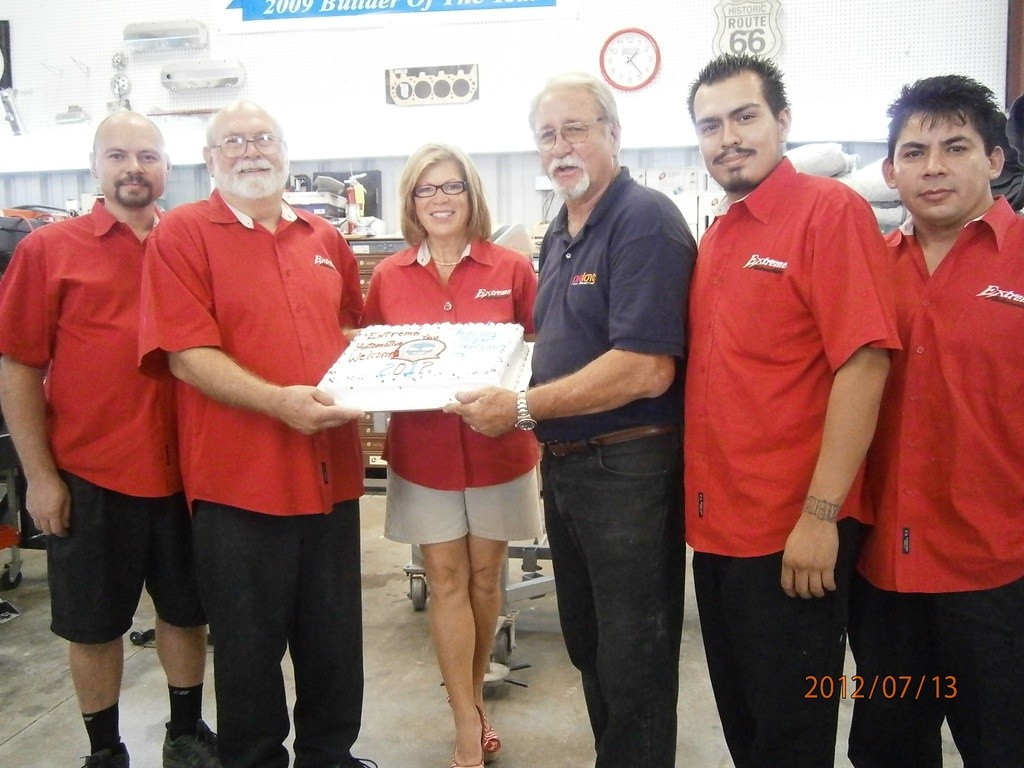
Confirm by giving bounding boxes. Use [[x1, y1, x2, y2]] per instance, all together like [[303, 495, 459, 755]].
[[162, 720, 224, 768], [79, 744, 130, 768]]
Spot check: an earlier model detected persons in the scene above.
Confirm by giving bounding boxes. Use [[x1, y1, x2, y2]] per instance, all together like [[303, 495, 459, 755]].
[[438, 71, 701, 768], [842, 75, 1023, 768], [0, 110, 230, 767], [681, 54, 904, 767], [136, 100, 383, 768], [356, 140, 546, 766]]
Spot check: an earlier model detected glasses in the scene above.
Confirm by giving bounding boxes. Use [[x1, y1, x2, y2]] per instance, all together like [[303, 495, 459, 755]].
[[533, 117, 607, 152], [208, 133, 289, 158], [413, 181, 468, 198]]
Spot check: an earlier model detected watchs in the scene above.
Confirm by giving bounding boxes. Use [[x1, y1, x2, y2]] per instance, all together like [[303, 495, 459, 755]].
[[514, 390, 538, 433]]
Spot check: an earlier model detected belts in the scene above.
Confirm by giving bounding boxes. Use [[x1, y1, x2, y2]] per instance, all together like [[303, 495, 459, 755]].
[[545, 423, 683, 457]]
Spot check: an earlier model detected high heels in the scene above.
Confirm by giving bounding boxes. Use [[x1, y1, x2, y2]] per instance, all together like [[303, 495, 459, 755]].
[[446, 691, 501, 762], [449, 704, 486, 768]]
